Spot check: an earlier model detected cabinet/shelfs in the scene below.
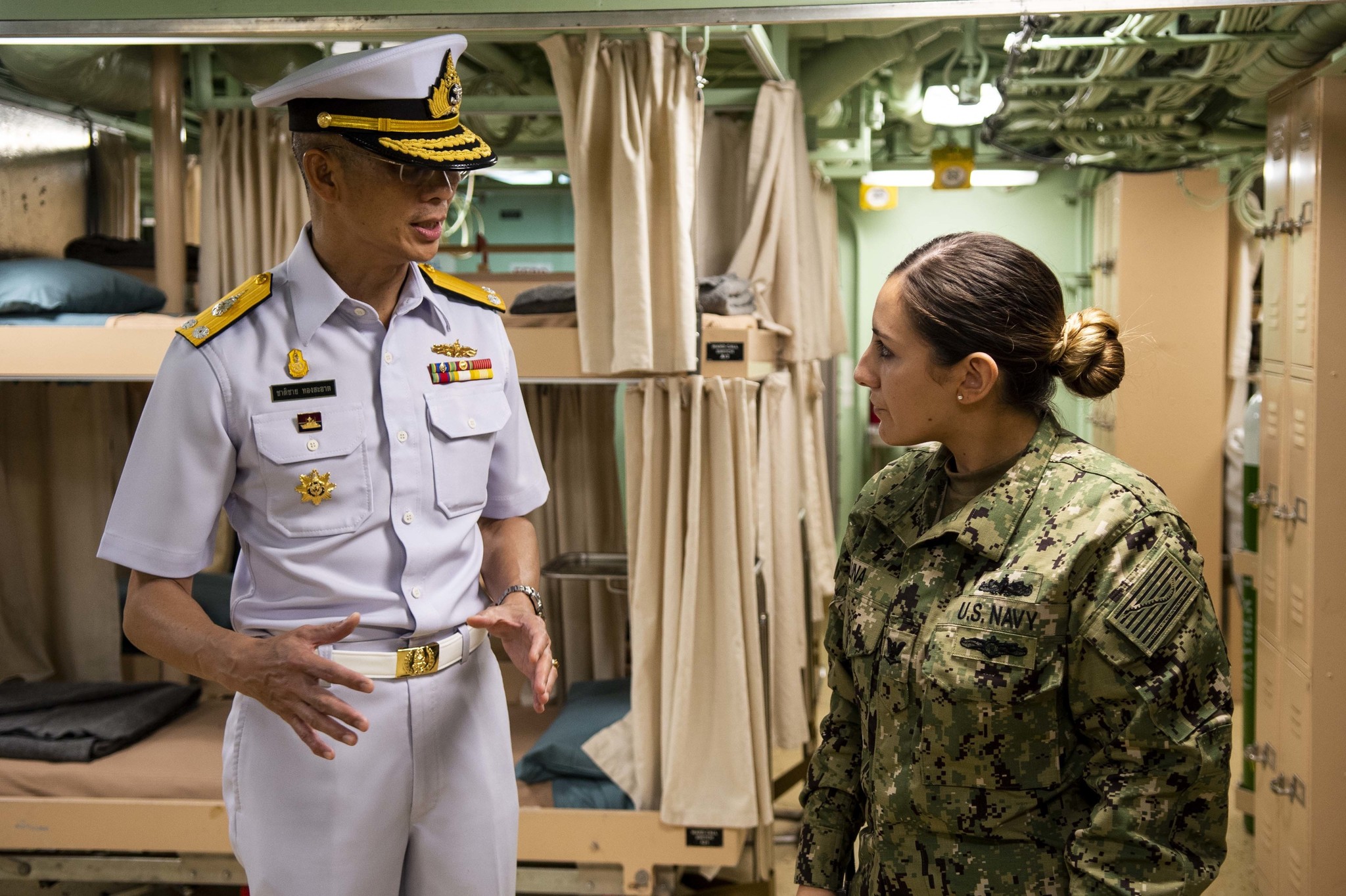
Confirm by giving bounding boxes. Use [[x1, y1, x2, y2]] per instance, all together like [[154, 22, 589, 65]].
[[1252, 60, 1346, 896], [1091, 168, 1226, 630]]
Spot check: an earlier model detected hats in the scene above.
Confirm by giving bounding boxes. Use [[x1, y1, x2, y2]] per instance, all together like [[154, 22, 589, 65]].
[[251, 33, 498, 169]]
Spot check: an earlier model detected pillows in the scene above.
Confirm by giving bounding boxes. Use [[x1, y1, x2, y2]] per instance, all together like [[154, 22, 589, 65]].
[[509, 281, 578, 315], [1, 254, 167, 313], [517, 677, 635, 809], [190, 570, 235, 629]]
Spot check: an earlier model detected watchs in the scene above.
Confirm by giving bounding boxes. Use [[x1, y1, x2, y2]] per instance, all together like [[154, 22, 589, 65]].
[[495, 584, 546, 621]]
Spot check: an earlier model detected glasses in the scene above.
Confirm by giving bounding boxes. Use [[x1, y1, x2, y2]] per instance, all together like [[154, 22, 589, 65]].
[[319, 145, 471, 185]]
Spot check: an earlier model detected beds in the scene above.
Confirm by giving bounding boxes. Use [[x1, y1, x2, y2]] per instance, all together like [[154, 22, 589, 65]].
[[1, 265, 790, 895]]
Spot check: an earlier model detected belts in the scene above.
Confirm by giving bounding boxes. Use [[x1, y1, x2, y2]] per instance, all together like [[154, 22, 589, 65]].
[[313, 624, 489, 679]]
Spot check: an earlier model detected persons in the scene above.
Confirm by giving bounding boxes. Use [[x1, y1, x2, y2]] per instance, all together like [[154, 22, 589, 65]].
[[97, 32, 559, 895], [793, 230, 1234, 896]]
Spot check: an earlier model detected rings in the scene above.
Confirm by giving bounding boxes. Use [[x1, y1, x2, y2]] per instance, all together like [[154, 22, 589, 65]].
[[552, 659, 559, 668]]
